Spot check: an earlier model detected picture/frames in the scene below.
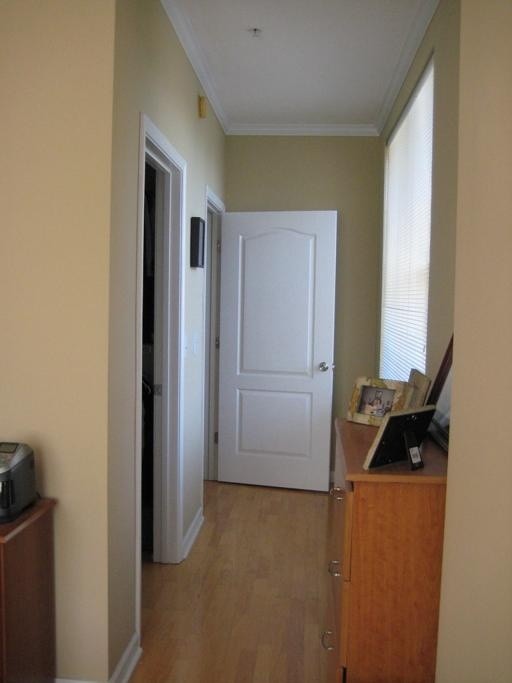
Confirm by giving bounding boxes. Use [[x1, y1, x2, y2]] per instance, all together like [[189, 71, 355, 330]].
[[404, 368, 432, 409], [346, 376, 409, 427], [362, 404, 437, 471]]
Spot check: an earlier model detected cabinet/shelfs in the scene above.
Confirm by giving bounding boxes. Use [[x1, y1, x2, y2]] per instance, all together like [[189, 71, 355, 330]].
[[0, 497, 58, 683], [321, 418, 448, 683]]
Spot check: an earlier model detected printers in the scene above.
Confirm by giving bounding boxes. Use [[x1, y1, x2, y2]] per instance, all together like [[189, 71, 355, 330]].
[[0, 441, 36, 522]]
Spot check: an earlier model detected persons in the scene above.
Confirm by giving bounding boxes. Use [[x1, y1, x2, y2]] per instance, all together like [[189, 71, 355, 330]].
[[368, 397, 392, 418]]
